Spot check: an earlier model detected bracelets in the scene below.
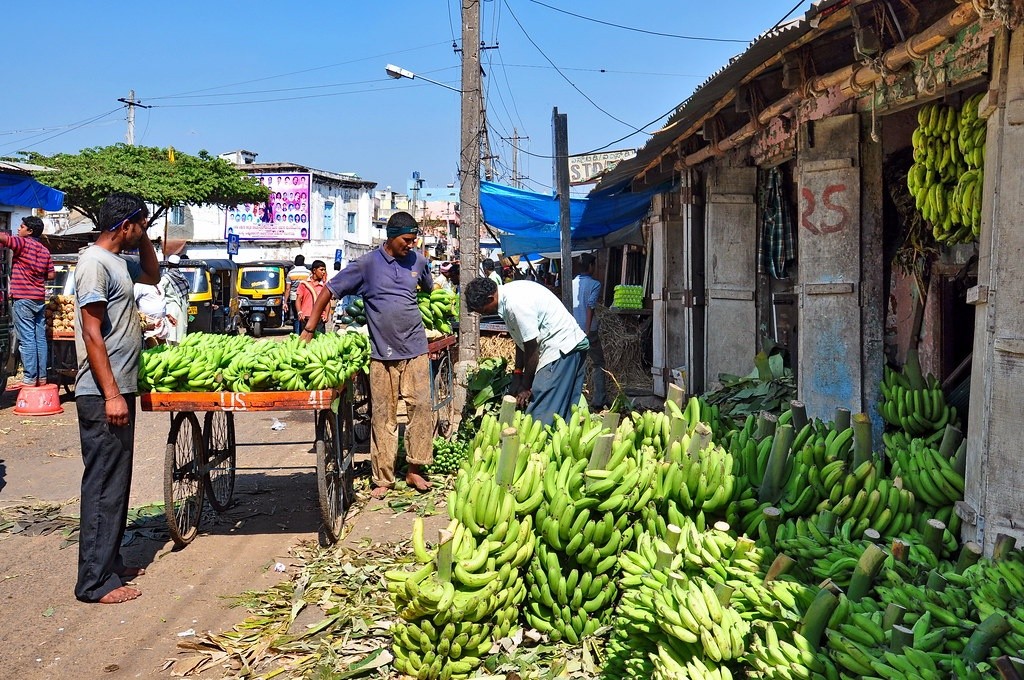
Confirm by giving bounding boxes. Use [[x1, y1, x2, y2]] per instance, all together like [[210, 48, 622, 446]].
[[105, 393, 119, 401], [514, 370, 521, 374], [298, 311, 302, 315], [304, 328, 315, 333]]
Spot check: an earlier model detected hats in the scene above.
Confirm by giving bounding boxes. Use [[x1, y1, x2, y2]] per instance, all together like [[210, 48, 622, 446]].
[[168, 255, 180, 264]]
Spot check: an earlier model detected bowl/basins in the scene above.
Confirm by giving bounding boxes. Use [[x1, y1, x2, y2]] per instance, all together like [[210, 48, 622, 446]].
[[11, 382, 64, 416]]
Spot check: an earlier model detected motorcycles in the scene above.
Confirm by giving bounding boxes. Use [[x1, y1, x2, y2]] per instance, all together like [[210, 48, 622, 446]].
[[234, 260, 295, 338], [159, 258, 240, 337]]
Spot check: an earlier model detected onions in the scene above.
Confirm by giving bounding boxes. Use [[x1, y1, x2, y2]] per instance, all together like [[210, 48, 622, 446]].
[[46, 295, 145, 332]]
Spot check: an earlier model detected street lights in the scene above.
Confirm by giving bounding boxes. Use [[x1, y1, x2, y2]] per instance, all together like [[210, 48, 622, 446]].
[[384, 63, 482, 432]]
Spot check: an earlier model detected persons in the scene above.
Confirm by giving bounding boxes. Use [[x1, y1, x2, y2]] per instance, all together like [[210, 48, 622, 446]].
[[464, 277, 590, 431], [296, 260, 331, 339], [435, 262, 460, 293], [328, 262, 341, 279], [521, 268, 535, 281], [73, 192, 161, 603], [301, 228, 307, 237], [538, 270, 545, 282], [230, 177, 307, 222], [283, 255, 312, 334], [483, 258, 502, 286], [301, 211, 434, 496], [572, 253, 611, 408], [0, 216, 55, 388], [505, 270, 513, 282], [134, 254, 189, 349]]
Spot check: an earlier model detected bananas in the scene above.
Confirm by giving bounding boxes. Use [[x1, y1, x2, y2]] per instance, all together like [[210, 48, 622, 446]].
[[138, 288, 1024, 680], [908, 91, 987, 246]]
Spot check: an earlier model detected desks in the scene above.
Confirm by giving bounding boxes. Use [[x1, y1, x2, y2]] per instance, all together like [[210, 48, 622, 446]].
[[611, 306, 653, 335]]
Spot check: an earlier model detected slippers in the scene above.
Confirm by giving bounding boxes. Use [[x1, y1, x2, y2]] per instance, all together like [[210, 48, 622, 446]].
[[5, 381, 35, 391], [36, 377, 46, 386]]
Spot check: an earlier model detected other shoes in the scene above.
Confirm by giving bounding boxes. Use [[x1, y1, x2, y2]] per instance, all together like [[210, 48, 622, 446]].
[[592, 402, 612, 410]]
